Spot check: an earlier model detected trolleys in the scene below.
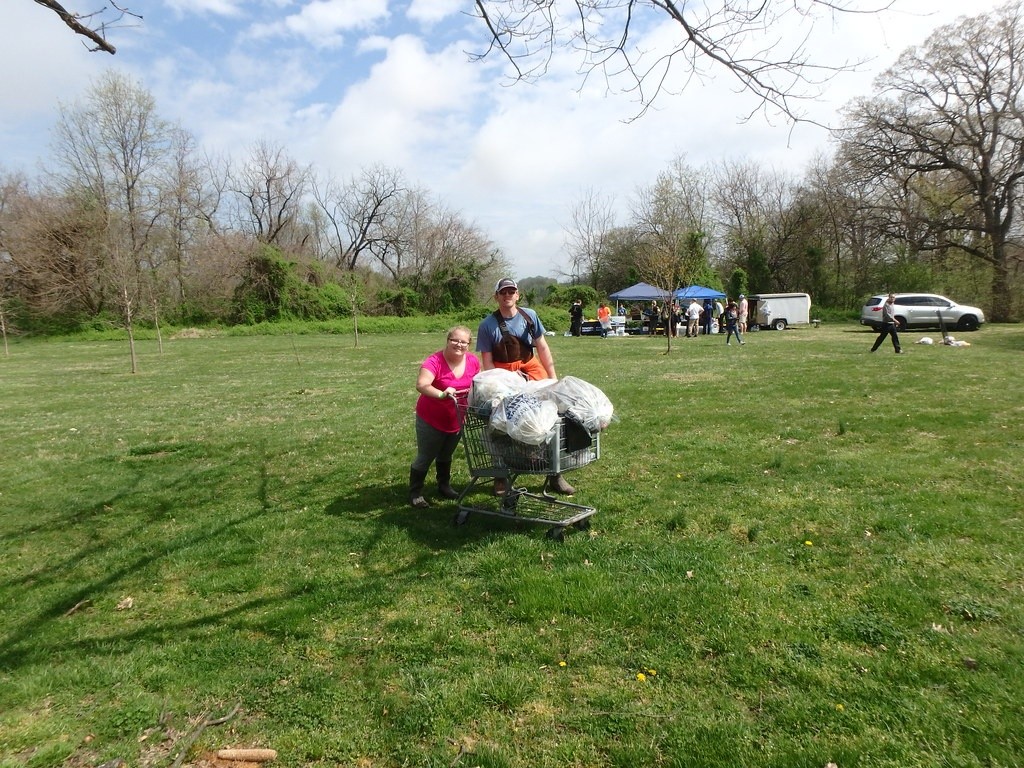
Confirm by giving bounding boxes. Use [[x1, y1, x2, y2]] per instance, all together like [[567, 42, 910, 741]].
[[447, 374, 600, 542]]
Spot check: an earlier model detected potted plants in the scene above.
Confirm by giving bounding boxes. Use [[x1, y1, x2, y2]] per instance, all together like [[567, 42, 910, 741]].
[[626, 320, 644, 335]]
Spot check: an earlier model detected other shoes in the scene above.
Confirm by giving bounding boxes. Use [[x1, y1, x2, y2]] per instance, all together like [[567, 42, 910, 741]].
[[899, 350, 904, 353], [494, 478, 506, 494], [727, 342, 731, 345], [740, 341, 745, 345], [551, 476, 575, 494]]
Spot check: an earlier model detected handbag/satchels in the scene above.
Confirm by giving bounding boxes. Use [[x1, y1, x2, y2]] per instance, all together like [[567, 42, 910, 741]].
[[662, 308, 669, 320], [573, 307, 578, 316], [565, 410, 592, 452]]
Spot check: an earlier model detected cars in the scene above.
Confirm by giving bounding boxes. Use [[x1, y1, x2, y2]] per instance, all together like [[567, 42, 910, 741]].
[[860, 293, 986, 332], [580, 320, 602, 335]]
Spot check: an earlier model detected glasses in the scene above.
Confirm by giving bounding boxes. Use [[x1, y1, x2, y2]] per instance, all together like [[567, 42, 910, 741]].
[[500, 289, 516, 295], [448, 338, 470, 347]]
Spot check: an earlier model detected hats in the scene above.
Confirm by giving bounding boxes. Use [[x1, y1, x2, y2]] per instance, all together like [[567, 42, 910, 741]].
[[739, 295, 744, 298], [496, 277, 518, 294]]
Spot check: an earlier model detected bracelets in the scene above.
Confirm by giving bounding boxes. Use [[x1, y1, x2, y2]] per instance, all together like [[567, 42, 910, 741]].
[[440, 392, 444, 400]]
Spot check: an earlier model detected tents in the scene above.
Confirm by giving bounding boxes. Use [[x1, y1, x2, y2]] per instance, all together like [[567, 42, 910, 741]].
[[674, 285, 728, 305], [609, 282, 677, 316]]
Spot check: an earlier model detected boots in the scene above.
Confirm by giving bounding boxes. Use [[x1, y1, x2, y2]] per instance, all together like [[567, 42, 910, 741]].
[[436, 461, 459, 498], [409, 464, 428, 507]]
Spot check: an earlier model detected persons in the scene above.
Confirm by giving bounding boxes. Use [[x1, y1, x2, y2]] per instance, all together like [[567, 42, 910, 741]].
[[475, 278, 576, 495], [649, 301, 677, 338], [714, 295, 748, 345], [618, 303, 626, 315], [870, 292, 902, 354], [597, 303, 611, 339], [569, 300, 582, 337], [408, 325, 481, 509], [686, 299, 713, 337]]
